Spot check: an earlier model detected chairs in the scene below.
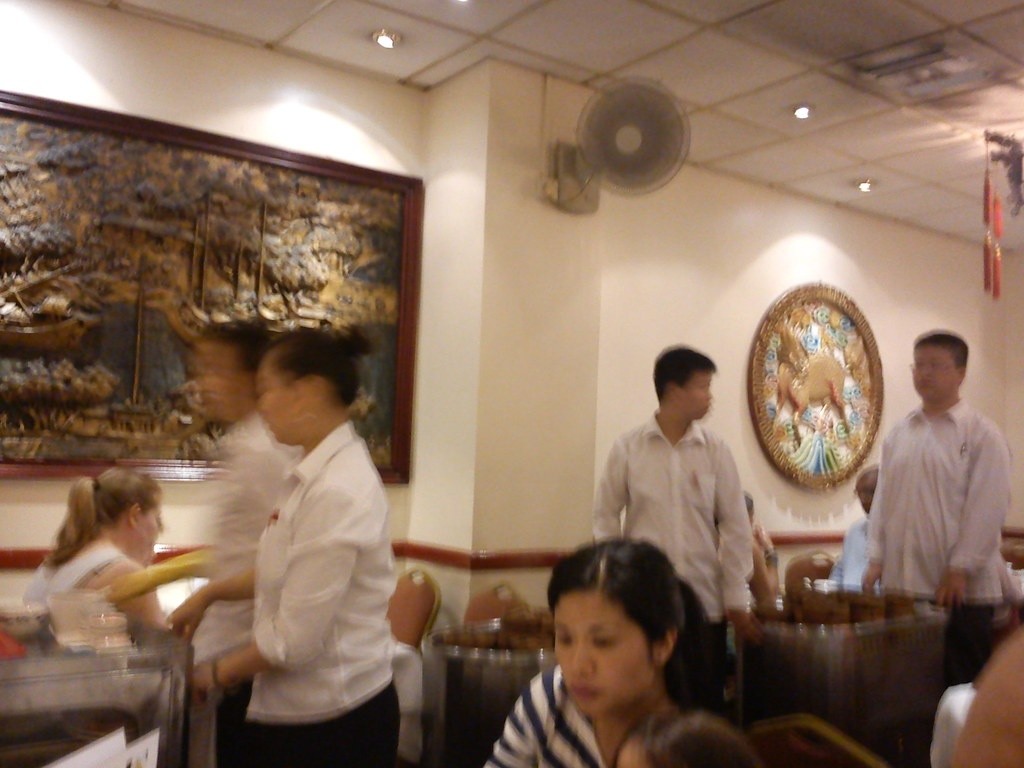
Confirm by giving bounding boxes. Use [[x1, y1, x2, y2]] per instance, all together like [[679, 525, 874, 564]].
[[383, 567, 442, 654]]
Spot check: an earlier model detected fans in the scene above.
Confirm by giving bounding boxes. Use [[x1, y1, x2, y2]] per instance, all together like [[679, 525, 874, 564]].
[[543, 78, 690, 214]]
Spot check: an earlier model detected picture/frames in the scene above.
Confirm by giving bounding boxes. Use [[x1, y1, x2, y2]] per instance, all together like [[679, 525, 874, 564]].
[[0, 90, 422, 485]]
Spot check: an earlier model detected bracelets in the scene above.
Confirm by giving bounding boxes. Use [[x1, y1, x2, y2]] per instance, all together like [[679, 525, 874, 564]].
[[212, 661, 226, 692]]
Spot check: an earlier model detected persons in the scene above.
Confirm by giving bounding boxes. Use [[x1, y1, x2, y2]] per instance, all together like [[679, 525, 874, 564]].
[[24, 466, 171, 636], [861, 334, 1011, 684], [168, 325, 402, 768], [97, 320, 304, 768], [594, 349, 766, 714], [931, 601, 1024, 768], [716, 495, 779, 677], [828, 467, 879, 587], [484, 537, 769, 768]]
